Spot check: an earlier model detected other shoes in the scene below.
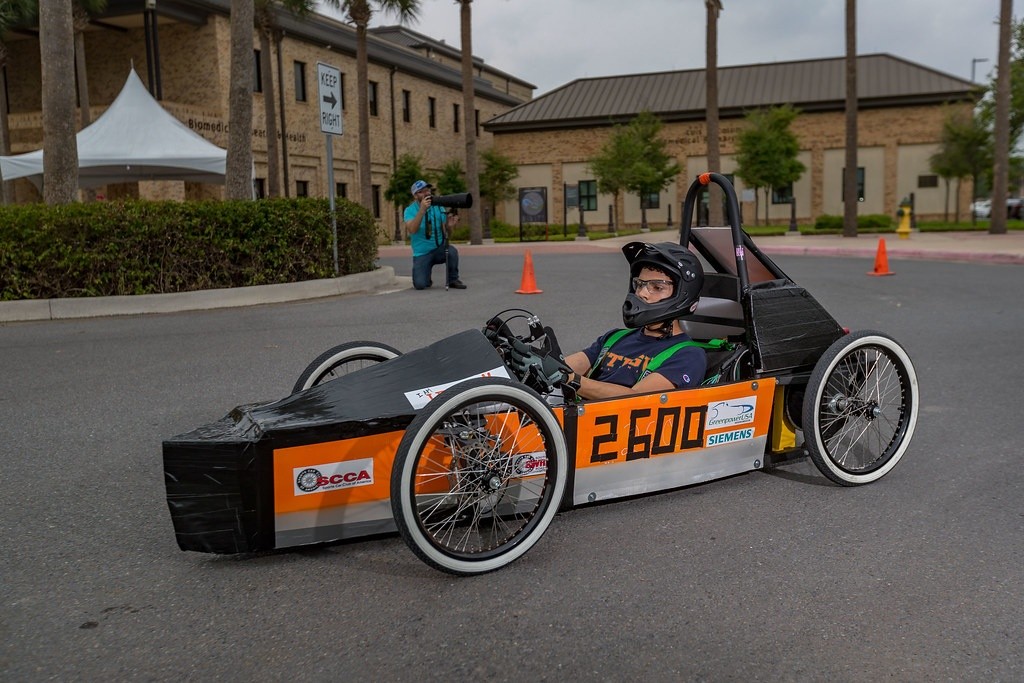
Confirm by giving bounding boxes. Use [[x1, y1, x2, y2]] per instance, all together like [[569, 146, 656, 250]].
[[427, 272, 433, 288], [446, 279, 466, 289]]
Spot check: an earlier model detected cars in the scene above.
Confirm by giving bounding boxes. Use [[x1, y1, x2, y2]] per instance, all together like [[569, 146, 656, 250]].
[[970, 196, 1024, 220]]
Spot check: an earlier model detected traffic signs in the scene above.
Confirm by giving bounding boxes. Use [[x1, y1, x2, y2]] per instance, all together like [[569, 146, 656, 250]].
[[316, 61, 344, 136]]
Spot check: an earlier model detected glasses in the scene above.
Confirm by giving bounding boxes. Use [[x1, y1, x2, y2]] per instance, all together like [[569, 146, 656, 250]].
[[417, 186, 431, 193], [632, 275, 674, 294]]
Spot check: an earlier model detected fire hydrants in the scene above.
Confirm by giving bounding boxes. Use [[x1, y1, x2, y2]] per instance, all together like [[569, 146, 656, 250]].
[[894, 195, 915, 239]]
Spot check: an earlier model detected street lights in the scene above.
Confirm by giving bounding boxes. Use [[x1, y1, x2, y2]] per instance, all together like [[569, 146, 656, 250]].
[[970, 57, 990, 84]]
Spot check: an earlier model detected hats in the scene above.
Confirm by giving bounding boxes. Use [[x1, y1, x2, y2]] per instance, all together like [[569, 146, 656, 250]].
[[410, 180, 432, 196]]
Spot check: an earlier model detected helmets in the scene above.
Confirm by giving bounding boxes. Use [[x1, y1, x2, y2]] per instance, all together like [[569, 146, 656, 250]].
[[622, 241, 704, 329]]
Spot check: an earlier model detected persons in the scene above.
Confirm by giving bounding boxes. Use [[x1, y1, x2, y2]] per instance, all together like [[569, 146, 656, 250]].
[[482, 241, 705, 401], [403, 180, 467, 290]]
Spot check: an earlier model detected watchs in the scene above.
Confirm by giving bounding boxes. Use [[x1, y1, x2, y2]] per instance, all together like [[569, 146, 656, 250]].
[[567, 373, 581, 392]]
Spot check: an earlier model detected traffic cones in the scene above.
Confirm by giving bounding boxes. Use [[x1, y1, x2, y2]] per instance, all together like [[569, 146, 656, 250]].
[[866, 238, 896, 276], [514, 248, 542, 296]]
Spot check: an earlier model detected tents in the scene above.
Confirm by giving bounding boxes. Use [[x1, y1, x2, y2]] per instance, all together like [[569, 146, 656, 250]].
[[0, 58, 256, 202]]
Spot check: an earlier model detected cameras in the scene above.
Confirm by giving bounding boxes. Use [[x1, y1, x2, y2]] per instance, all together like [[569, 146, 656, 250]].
[[426, 192, 473, 208]]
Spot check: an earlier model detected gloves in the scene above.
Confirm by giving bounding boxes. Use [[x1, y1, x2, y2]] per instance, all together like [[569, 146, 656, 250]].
[[507, 326, 573, 394], [482, 318, 544, 361]]
